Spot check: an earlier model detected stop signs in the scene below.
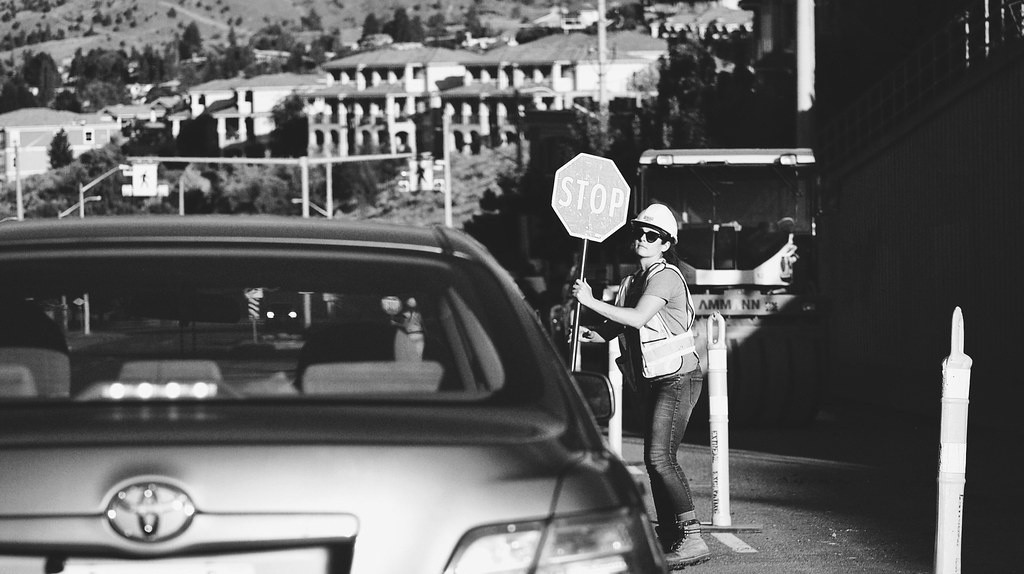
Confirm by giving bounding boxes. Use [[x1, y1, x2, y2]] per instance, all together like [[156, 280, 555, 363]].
[[552, 153, 632, 244]]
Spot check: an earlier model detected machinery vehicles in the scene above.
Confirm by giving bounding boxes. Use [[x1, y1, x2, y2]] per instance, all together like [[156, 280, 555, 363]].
[[629, 148, 828, 431]]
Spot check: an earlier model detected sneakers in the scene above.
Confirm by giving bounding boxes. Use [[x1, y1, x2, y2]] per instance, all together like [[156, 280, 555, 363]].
[[654, 526, 684, 552], [663, 519, 714, 569]]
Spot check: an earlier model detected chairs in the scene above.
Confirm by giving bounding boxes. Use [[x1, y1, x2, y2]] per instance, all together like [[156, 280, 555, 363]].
[[296, 321, 422, 393], [0, 307, 74, 398]]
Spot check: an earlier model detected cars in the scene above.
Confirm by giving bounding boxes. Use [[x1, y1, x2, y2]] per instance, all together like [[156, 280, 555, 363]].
[[0, 213, 671, 574]]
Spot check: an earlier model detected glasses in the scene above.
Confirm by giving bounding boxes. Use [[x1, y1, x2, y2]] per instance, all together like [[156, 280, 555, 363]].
[[633, 226, 669, 243]]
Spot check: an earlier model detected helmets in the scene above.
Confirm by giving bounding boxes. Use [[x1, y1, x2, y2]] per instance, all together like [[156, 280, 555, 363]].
[[631, 204, 679, 245]]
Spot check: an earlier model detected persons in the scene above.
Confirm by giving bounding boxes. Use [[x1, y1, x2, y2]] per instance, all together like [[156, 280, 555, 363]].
[[566, 204, 714, 574]]
